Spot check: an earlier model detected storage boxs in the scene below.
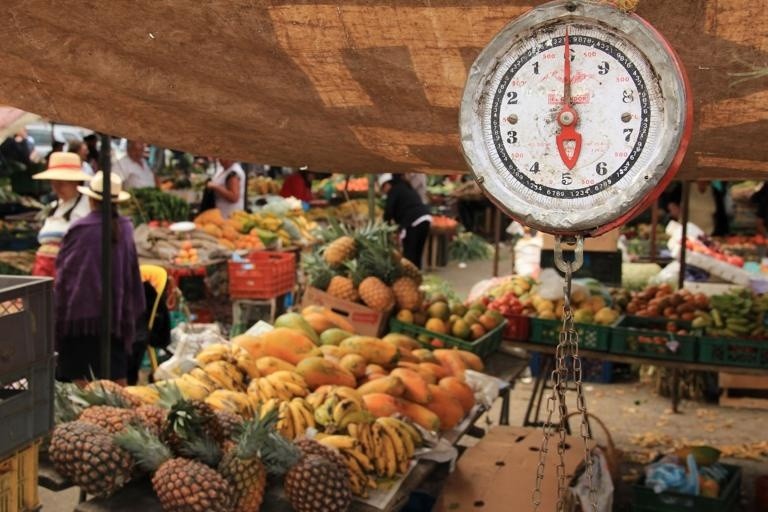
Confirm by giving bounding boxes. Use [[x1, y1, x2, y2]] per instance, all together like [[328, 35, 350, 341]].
[[0, 166, 510, 511], [477, 287, 768, 371], [534, 215, 627, 381]]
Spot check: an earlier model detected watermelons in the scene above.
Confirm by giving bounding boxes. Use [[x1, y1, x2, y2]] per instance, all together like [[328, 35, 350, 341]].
[[456, 0, 693, 512]]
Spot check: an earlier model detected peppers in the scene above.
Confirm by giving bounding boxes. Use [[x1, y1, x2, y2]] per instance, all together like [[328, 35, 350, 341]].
[[535, 289, 622, 325]]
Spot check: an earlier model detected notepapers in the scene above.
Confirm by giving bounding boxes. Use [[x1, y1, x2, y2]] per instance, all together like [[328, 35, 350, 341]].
[[305, 218, 424, 310]]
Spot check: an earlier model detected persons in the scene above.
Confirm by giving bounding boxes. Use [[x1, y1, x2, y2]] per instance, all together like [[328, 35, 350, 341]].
[[206, 158, 246, 214], [2, 123, 156, 197], [412, 172, 427, 203], [280, 166, 314, 211], [32, 152, 90, 277], [664, 202, 706, 257], [54, 170, 146, 382], [378, 172, 432, 270]]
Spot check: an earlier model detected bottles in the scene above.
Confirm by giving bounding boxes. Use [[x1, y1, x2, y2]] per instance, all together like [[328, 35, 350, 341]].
[[76, 170, 133, 203], [32, 151, 93, 183], [378, 173, 392, 191]]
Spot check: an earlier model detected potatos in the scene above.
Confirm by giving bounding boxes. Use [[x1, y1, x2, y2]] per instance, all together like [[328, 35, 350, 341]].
[[707, 288, 768, 337]]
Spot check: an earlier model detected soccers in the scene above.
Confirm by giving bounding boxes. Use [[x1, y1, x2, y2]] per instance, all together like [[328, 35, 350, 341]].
[[398, 291, 502, 349]]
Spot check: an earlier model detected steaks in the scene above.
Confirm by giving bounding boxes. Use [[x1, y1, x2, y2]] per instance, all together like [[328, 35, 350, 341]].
[[672, 444, 721, 466], [192, 209, 289, 251], [699, 475, 718, 499]]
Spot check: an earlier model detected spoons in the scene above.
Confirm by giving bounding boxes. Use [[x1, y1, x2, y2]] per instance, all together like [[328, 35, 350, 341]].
[[685, 240, 744, 266], [479, 291, 534, 339], [625, 283, 706, 354]]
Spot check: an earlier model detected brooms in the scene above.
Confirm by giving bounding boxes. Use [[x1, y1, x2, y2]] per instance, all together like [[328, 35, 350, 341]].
[[230, 210, 291, 246], [230, 305, 485, 431]]
